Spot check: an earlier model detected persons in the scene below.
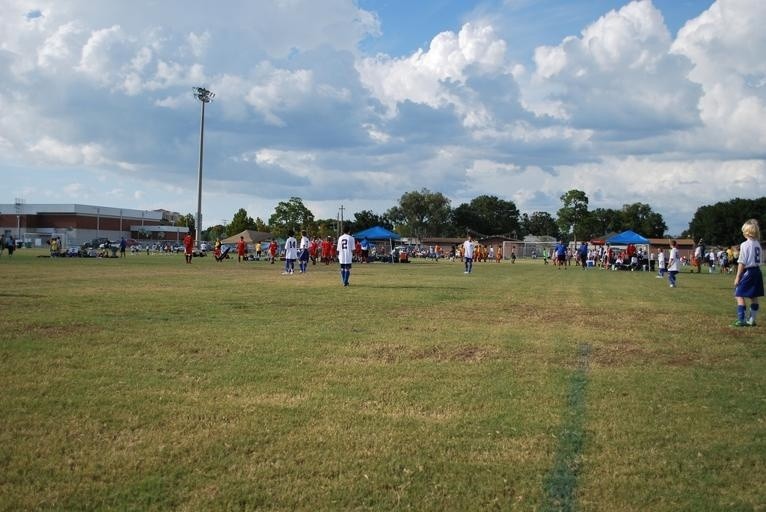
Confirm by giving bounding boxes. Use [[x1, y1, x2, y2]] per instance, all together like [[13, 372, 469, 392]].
[[6, 235, 15, 256], [657, 236, 731, 288], [355, 236, 370, 262], [201, 239, 222, 260], [119, 237, 126, 258], [412, 235, 502, 274], [282, 230, 337, 274], [543, 239, 644, 271], [730, 217, 766, 327], [336, 226, 356, 286], [51, 236, 60, 257], [130, 244, 180, 256], [236, 236, 277, 264], [510, 246, 516, 264], [183, 232, 193, 264]]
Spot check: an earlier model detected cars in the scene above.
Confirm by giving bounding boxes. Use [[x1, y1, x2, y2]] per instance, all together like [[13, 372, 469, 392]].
[[391, 246, 410, 256], [80, 236, 210, 253], [369, 243, 385, 255]]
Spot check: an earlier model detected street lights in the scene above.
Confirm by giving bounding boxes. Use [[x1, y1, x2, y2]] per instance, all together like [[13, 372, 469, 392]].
[[191, 86, 215, 248]]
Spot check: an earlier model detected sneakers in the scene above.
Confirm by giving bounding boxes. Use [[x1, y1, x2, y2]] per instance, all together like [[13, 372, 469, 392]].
[[281, 270, 306, 276], [343, 282, 350, 286], [543, 262, 587, 272], [464, 271, 473, 274], [655, 270, 757, 327]]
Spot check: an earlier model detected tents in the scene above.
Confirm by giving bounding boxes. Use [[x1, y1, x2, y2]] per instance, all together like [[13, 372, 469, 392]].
[[353, 226, 399, 264], [604, 229, 650, 273]]
